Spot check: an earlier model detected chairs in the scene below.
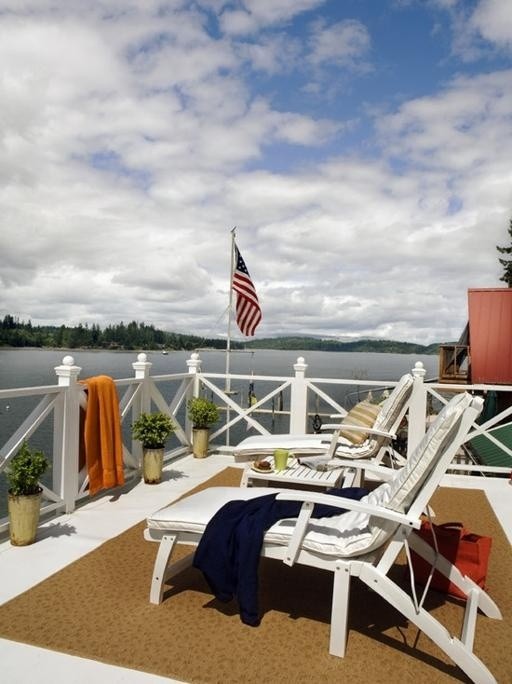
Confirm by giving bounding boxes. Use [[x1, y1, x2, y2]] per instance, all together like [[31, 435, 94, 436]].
[[227, 372, 418, 494], [142, 392, 503, 683]]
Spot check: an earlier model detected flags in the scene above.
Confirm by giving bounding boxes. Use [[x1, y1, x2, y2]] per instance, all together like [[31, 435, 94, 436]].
[[232, 239, 263, 337]]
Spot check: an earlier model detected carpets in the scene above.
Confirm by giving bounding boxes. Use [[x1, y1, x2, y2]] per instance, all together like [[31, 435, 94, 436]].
[[1, 465, 512, 682]]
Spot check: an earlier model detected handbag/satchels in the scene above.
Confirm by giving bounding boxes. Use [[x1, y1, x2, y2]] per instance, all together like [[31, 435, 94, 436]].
[[406, 519, 492, 603]]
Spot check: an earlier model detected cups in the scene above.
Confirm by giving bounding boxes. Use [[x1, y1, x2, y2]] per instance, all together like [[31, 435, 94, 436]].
[[273, 450, 295, 472]]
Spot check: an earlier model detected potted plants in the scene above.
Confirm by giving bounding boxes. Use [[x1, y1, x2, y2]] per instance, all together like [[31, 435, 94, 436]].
[[126, 411, 179, 485], [180, 393, 225, 460], [5, 440, 50, 549]]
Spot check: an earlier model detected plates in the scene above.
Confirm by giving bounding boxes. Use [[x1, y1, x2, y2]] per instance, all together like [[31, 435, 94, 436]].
[[251, 461, 274, 473]]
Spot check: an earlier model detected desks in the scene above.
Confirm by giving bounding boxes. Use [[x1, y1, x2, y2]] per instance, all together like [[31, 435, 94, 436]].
[[234, 453, 348, 501]]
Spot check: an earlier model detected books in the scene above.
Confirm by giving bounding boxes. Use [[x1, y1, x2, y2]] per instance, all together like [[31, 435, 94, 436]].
[[298, 455, 341, 471]]
